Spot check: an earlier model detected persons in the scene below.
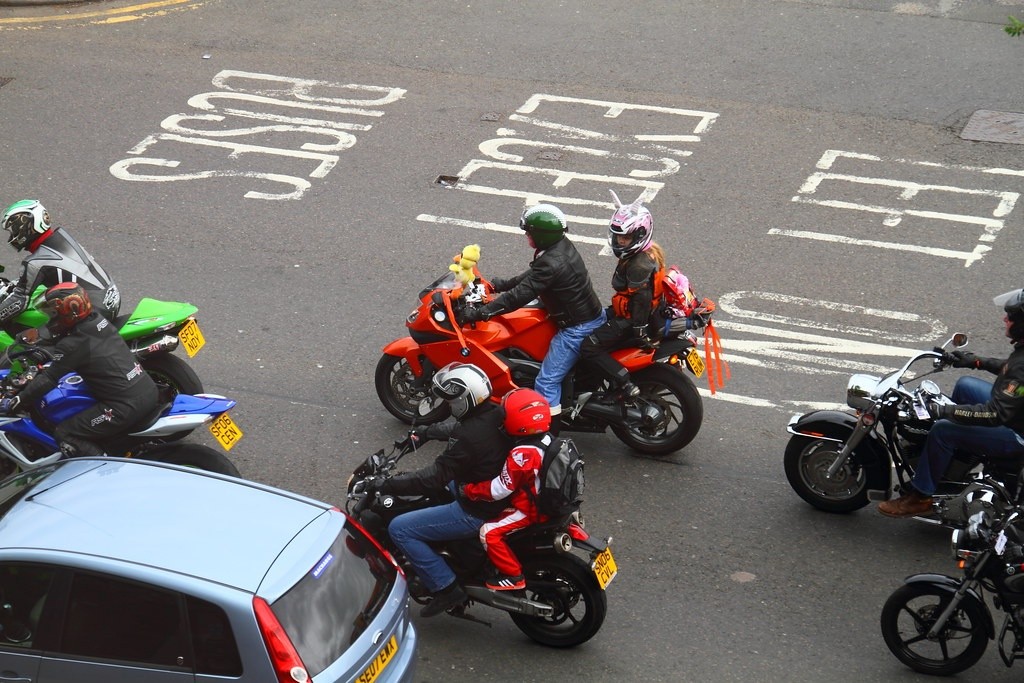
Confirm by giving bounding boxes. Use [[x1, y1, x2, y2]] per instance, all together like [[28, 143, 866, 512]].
[[0, 283, 160, 458], [360, 363, 514, 617], [579, 187, 666, 406], [0, 198, 121, 324], [458, 390, 556, 589], [460, 204, 608, 439], [878, 288, 1024, 517]]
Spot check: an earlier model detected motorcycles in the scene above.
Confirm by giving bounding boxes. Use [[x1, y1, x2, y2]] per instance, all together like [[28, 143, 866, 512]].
[[0, 261, 207, 443], [784, 332, 1024, 529], [345, 396, 619, 649], [879, 460, 1024, 677], [374, 263, 730, 455], [0, 326, 243, 482]]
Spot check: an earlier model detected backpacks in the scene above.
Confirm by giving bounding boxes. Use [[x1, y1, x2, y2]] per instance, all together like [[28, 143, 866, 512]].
[[521, 433, 583, 518], [662, 265, 699, 317]]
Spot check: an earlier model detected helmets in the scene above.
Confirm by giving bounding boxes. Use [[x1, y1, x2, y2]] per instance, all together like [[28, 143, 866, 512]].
[[503, 388, 551, 436], [42, 282, 93, 333], [523, 204, 566, 250], [609, 204, 654, 259], [433, 361, 493, 418], [1, 199, 51, 252], [1004, 290, 1024, 343]]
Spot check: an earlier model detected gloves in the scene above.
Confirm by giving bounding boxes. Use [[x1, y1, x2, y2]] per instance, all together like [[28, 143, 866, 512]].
[[927, 403, 945, 419], [459, 482, 466, 497], [489, 277, 507, 293], [413, 425, 428, 446], [7, 390, 32, 413], [950, 351, 980, 370], [365, 479, 385, 496], [457, 307, 480, 329], [635, 324, 654, 354]]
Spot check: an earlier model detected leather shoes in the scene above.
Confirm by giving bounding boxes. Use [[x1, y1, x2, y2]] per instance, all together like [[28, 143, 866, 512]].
[[878, 494, 933, 517]]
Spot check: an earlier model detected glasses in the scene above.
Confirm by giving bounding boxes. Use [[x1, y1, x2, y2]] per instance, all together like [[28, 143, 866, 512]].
[[610, 223, 627, 234], [519, 218, 526, 229]]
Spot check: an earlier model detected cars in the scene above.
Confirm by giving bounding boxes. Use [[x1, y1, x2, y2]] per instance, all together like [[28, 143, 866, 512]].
[[0, 454, 417, 683]]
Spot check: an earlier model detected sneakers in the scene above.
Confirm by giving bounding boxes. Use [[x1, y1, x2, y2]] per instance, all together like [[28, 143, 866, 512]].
[[485, 574, 526, 590], [601, 381, 640, 404], [420, 584, 467, 616]]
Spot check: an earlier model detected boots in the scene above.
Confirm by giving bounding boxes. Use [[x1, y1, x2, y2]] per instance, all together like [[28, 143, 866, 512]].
[[549, 404, 562, 437]]
